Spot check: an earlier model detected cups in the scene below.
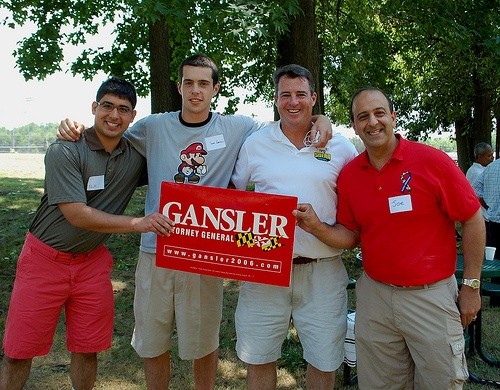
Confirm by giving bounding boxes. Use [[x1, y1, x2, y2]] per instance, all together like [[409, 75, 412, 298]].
[[485, 247, 496, 261]]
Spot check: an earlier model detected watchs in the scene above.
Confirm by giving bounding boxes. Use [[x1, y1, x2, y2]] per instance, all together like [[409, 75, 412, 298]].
[[461, 278, 481, 290]]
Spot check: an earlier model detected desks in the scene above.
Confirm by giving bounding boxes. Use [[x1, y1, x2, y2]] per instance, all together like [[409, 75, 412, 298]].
[[356, 250, 500, 278]]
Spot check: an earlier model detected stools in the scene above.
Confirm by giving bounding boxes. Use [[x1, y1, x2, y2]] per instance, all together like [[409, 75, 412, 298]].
[[475, 280, 500, 367]]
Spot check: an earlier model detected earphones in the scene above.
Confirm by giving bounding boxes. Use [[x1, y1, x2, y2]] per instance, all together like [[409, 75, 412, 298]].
[[313, 140, 318, 144]]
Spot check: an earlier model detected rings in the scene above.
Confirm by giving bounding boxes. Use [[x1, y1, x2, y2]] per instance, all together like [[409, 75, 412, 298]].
[[471, 315, 478, 321]]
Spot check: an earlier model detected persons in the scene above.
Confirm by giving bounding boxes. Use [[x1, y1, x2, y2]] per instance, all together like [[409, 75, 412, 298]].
[[466, 142, 500, 262], [0, 78, 176, 390], [55, 52, 335, 390], [290, 85, 488, 390], [227, 63, 360, 390]]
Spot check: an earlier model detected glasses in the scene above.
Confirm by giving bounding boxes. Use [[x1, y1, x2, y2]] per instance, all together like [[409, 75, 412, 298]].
[[96, 101, 134, 113]]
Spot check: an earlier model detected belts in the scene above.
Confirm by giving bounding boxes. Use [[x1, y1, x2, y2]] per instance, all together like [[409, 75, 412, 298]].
[[292, 256, 326, 265]]
